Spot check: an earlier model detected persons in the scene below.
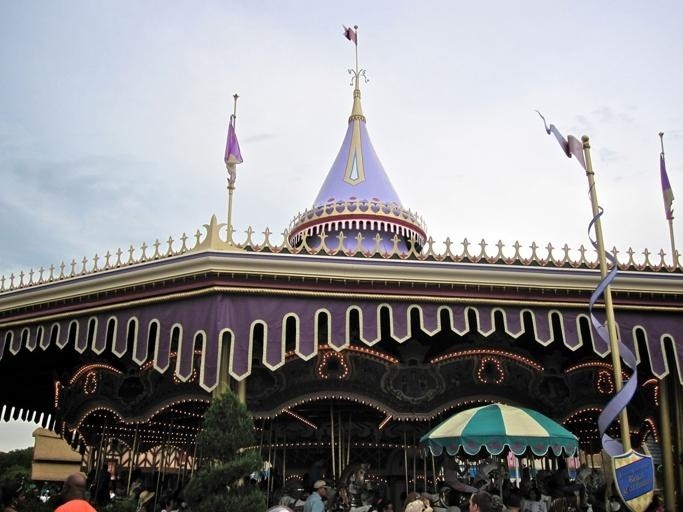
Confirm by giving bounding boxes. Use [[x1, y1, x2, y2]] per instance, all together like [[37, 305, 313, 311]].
[[93, 463, 679, 512], [0, 481, 64, 511], [52, 472, 98, 511]]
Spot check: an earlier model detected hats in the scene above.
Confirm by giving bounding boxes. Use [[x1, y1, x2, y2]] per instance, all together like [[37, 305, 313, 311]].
[[404, 499, 433, 512], [312, 479, 331, 489]]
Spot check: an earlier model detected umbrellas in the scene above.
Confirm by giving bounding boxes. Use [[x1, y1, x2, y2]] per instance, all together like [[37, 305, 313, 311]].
[[419, 401, 580, 499]]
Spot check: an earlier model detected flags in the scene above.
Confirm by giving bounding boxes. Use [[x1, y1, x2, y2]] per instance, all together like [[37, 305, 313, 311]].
[[657, 152, 675, 220], [224, 116, 242, 185]]
